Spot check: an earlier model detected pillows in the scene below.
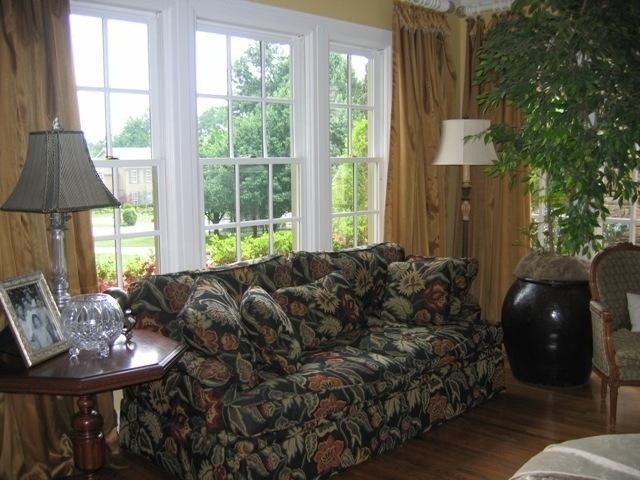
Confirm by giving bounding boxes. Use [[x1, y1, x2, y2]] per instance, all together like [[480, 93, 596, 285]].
[[626, 292, 640, 332]]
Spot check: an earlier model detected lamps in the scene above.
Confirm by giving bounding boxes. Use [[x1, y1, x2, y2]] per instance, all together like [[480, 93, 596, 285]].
[[0, 114, 125, 314], [432, 119, 500, 261]]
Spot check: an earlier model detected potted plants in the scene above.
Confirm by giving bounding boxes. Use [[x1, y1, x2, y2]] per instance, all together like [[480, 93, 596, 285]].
[[461, 0, 640, 391]]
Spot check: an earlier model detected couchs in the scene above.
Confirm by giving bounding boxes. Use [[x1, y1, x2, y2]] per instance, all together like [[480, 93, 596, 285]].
[[118, 242, 505, 479]]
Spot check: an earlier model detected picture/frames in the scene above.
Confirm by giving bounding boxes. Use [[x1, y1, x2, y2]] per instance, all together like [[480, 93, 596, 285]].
[[0, 270, 71, 369]]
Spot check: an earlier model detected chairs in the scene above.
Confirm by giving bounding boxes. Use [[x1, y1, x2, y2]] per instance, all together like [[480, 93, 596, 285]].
[[588, 241, 640, 434]]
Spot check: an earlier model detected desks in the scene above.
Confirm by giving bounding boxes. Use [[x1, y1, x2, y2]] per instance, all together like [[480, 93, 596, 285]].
[[0, 318, 188, 480]]
